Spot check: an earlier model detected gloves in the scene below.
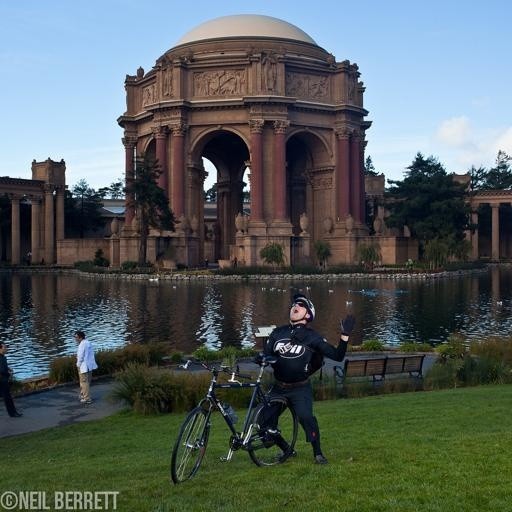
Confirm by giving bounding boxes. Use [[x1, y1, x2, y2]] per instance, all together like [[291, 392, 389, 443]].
[[341, 314, 355, 336]]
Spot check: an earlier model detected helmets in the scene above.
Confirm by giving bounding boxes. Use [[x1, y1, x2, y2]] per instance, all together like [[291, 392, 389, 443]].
[[294, 297, 316, 321]]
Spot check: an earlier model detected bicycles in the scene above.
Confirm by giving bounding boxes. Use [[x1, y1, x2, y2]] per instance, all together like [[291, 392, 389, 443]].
[[170, 353, 299, 486]]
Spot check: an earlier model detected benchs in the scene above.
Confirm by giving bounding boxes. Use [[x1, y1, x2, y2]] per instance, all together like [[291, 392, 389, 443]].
[[331, 354, 426, 384]]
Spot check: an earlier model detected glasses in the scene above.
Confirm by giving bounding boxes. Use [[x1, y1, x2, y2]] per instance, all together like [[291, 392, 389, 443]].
[[292, 303, 304, 307]]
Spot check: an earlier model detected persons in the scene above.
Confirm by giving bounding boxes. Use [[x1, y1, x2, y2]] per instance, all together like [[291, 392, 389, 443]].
[[233, 255, 238, 268], [205, 256, 209, 267], [103, 261, 109, 267], [27, 251, 32, 264], [74, 331, 99, 403], [0, 339, 24, 417], [143, 50, 327, 104], [254, 296, 357, 463]]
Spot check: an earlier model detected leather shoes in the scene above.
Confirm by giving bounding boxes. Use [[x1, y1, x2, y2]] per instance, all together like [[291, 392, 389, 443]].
[[10, 411, 24, 417]]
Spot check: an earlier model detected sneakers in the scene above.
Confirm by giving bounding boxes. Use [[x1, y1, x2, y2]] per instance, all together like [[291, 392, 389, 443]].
[[80, 398, 91, 404], [278, 450, 297, 459], [313, 454, 328, 464]]
